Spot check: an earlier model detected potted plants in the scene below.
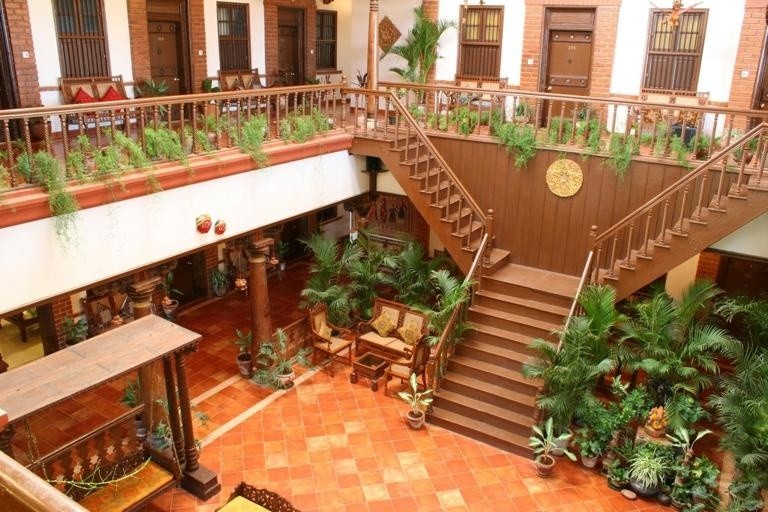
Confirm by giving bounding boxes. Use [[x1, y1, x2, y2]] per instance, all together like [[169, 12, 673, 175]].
[[254, 327, 316, 390], [231, 324, 254, 377], [197, 78, 223, 123], [717, 127, 767, 166], [156, 272, 186, 318], [528, 416, 577, 478], [134, 78, 169, 130], [378, 5, 457, 119], [26, 103, 51, 140], [353, 68, 368, 127], [396, 374, 436, 428], [597, 281, 763, 512], [210, 269, 229, 298], [521, 315, 604, 457], [580, 439, 602, 468], [274, 239, 288, 270]]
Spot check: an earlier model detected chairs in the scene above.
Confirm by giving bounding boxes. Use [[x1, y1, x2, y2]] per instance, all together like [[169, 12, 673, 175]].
[[307, 295, 435, 397]]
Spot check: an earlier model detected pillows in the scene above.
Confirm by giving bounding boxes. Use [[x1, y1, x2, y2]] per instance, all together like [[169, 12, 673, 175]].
[[99, 85, 129, 113], [230, 79, 247, 100], [247, 78, 266, 99], [71, 87, 99, 115], [641, 105, 662, 123], [458, 84, 476, 104], [675, 107, 697, 125]]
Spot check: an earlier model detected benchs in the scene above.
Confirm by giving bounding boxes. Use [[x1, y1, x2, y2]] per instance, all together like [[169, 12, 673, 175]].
[[22, 399, 183, 512], [436, 73, 508, 124], [222, 237, 282, 289], [622, 88, 710, 146], [79, 287, 136, 336], [217, 68, 270, 115], [57, 73, 138, 140]]
